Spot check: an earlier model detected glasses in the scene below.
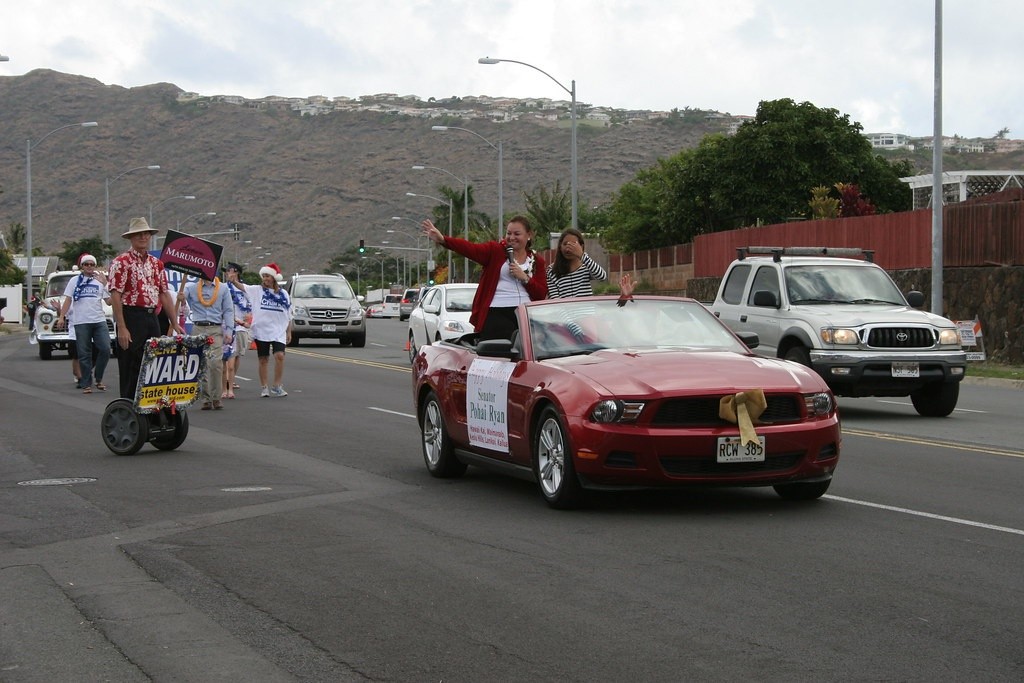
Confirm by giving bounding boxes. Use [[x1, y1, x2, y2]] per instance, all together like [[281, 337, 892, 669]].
[[83, 262, 95, 267]]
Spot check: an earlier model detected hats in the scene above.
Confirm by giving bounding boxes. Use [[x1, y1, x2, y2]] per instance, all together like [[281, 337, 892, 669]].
[[31, 296, 36, 301], [78, 253, 97, 268], [121, 217, 159, 239], [259, 263, 283, 282], [221, 261, 243, 275]]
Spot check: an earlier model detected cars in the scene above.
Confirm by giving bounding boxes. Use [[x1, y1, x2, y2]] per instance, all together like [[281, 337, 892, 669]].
[[417, 287, 433, 305], [412, 294, 842, 511], [382, 294, 403, 319], [34, 269, 117, 359], [399, 289, 420, 322], [366, 303, 392, 318], [407, 282, 482, 360]]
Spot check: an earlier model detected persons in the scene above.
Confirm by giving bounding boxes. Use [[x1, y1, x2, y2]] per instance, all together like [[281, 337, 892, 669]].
[[618, 273, 681, 342], [94, 272, 190, 336], [108, 216, 186, 401], [546, 228, 607, 346], [421, 216, 548, 346], [27, 253, 112, 394], [183, 261, 292, 410]]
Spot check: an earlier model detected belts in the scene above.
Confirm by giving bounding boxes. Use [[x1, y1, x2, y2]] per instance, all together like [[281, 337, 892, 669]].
[[194, 321, 221, 326], [126, 306, 155, 314]]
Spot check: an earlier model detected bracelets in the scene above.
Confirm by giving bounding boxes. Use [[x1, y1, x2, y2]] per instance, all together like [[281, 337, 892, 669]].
[[521, 274, 530, 286]]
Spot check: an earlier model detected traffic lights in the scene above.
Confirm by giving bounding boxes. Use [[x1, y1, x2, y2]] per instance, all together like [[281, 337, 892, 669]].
[[429, 272, 434, 283], [359, 240, 364, 253]]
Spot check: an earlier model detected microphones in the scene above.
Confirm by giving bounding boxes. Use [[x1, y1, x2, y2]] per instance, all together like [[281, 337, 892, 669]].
[[506, 244, 515, 264]]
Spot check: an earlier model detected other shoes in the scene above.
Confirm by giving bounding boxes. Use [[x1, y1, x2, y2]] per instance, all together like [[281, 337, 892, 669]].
[[201, 402, 212, 409], [221, 392, 235, 399], [213, 400, 225, 408], [83, 387, 92, 393], [94, 381, 107, 391]]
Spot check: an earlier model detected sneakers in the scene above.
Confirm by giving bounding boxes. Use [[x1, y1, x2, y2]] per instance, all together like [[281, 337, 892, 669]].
[[271, 385, 288, 397], [261, 385, 270, 398]]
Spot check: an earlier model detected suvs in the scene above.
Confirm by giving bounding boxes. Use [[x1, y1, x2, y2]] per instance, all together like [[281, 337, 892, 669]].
[[288, 272, 368, 349]]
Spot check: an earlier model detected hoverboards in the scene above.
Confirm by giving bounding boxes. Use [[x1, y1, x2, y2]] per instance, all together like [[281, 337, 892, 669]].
[[101, 398, 190, 457]]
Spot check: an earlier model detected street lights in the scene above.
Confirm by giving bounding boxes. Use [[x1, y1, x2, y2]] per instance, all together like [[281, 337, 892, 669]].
[[26, 121, 98, 323], [478, 57, 579, 232], [176, 212, 216, 233], [340, 192, 453, 304], [432, 125, 504, 243], [412, 165, 469, 283], [149, 195, 196, 252], [104, 165, 161, 278]]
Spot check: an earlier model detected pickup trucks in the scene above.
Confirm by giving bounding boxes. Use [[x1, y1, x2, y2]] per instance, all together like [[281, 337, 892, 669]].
[[700, 243, 969, 419]]
